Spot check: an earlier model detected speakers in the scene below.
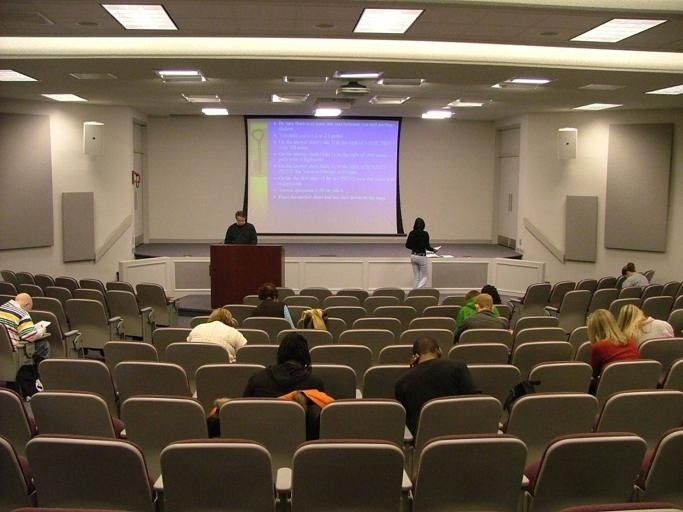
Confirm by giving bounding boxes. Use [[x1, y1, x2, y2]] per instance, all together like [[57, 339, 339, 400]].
[[557, 127, 578, 162], [82, 121, 104, 158]]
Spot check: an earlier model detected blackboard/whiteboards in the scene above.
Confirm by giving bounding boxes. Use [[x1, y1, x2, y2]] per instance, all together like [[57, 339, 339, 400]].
[[142, 116, 498, 245]]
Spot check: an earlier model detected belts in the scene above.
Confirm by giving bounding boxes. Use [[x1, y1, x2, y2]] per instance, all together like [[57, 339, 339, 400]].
[[411, 252, 426, 256]]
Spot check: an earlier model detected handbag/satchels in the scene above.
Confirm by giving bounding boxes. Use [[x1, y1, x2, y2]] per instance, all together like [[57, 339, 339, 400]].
[[296, 309, 329, 330], [16, 364, 43, 396]]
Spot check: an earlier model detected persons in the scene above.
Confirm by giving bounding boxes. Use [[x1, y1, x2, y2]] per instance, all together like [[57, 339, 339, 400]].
[[249, 281, 296, 330], [187, 308, 248, 363], [405, 218, 437, 289], [0, 293, 51, 361], [454, 294, 509, 343], [456, 290, 500, 325], [617, 304, 674, 352], [621, 262, 650, 291], [481, 285, 501, 304], [243, 333, 324, 398], [579, 308, 639, 376], [395, 335, 477, 438], [225, 211, 257, 246]]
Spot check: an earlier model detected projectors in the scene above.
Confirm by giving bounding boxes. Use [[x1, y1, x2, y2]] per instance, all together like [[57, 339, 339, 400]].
[[332, 83, 372, 98]]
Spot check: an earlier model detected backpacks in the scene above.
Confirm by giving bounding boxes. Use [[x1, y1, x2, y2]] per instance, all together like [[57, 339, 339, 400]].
[[504, 380, 541, 410]]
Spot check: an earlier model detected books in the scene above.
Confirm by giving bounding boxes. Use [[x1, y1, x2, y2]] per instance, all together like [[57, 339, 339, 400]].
[[426, 245, 442, 254], [34, 320, 51, 330]]
